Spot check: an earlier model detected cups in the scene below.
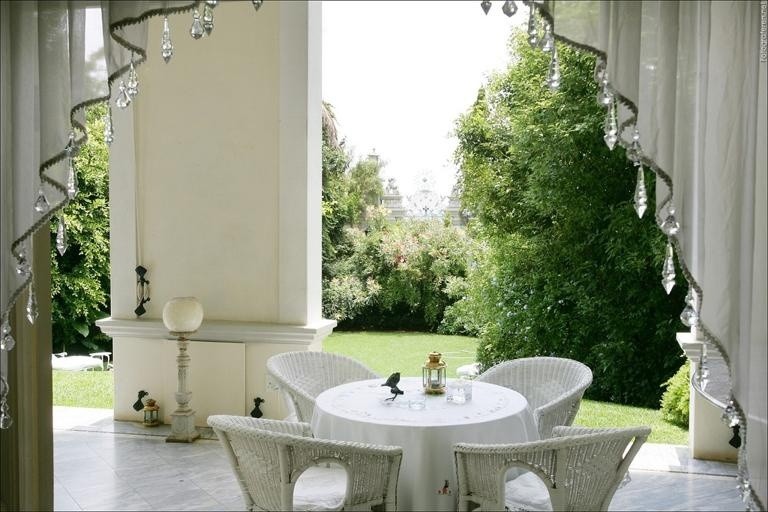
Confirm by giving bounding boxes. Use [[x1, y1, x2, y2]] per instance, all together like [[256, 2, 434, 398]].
[[446, 380, 473, 403], [407, 389, 426, 409]]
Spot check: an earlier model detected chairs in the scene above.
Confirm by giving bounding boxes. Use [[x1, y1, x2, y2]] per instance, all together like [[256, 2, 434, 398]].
[[207, 413, 403, 512], [472, 355, 593, 443], [452, 425, 651, 512], [264, 350, 380, 430]]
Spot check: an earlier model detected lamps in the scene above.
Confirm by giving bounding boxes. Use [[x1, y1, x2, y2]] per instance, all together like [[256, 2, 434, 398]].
[[161, 296, 205, 444]]
